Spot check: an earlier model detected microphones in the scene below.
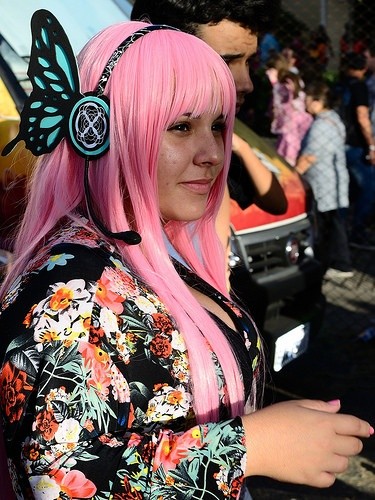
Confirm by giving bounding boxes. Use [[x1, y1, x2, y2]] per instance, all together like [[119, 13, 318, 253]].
[[83, 159, 142, 245]]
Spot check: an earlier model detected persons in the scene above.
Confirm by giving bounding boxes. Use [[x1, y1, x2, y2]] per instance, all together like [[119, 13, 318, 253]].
[[263, 0, 375, 274], [0, 20, 375, 500], [131, 0, 286, 260]]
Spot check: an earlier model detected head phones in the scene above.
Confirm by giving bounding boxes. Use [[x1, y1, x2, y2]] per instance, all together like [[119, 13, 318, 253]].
[[0, 9, 181, 159]]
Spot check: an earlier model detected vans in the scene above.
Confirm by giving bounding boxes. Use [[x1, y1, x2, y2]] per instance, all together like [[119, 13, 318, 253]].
[[0, 0, 319, 334]]
